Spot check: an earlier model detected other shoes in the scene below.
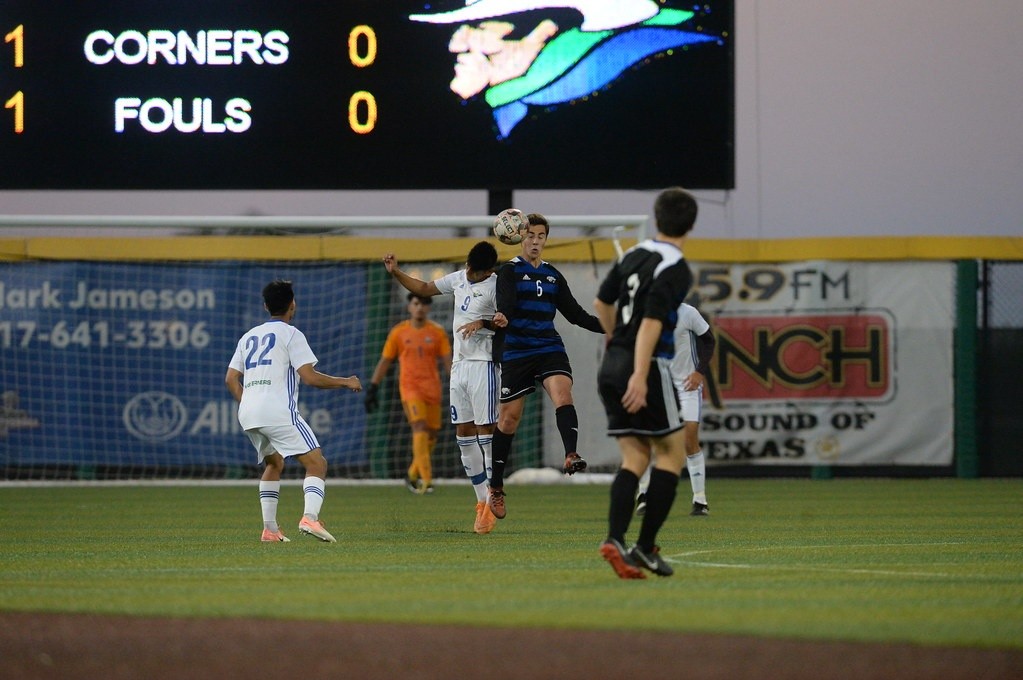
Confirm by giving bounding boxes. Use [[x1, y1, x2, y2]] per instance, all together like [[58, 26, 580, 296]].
[[406, 477, 419, 493], [426, 485, 434, 493]]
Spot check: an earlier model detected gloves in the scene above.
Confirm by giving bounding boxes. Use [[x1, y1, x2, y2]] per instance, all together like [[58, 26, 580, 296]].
[[364, 383, 378, 413]]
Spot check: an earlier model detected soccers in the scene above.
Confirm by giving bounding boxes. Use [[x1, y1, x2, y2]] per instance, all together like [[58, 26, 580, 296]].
[[493, 208, 531, 245]]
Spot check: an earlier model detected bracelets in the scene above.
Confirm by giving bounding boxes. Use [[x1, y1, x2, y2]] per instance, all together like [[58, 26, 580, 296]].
[[482, 320, 490, 328]]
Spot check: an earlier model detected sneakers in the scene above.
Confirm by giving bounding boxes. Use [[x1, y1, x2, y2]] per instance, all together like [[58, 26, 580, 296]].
[[636, 492, 647, 516], [474, 502, 487, 535], [689, 502, 710, 515], [628, 544, 674, 577], [478, 502, 497, 531], [488, 485, 507, 519], [563, 452, 587, 476], [600, 538, 647, 579], [261, 528, 290, 542], [299, 515, 337, 543]]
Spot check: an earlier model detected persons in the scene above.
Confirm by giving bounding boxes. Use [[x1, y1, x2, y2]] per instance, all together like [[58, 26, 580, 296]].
[[364, 292, 452, 494], [225, 281, 362, 544], [636, 303, 716, 515], [593, 189, 698, 580], [486, 213, 607, 520], [382, 241, 498, 534]]
